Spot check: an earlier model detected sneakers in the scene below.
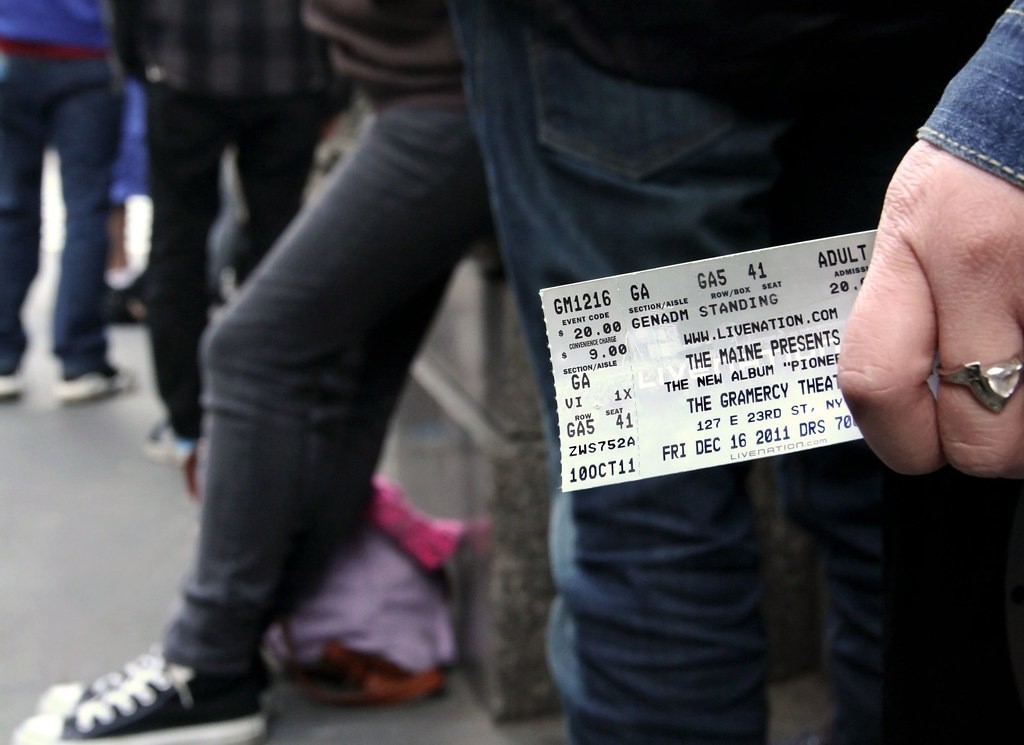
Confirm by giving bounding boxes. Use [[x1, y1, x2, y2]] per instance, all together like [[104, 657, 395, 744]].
[[12, 644, 279, 744], [139, 414, 195, 468], [1, 371, 24, 399], [58, 364, 126, 402]]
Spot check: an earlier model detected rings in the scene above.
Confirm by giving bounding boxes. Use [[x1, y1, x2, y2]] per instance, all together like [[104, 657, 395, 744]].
[[937, 355, 1024, 415]]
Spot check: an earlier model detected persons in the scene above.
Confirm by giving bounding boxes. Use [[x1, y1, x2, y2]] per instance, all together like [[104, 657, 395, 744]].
[[0, 0, 1024, 745]]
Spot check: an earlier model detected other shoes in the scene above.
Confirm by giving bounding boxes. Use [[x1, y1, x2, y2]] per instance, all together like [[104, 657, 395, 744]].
[[306, 688, 349, 706]]
